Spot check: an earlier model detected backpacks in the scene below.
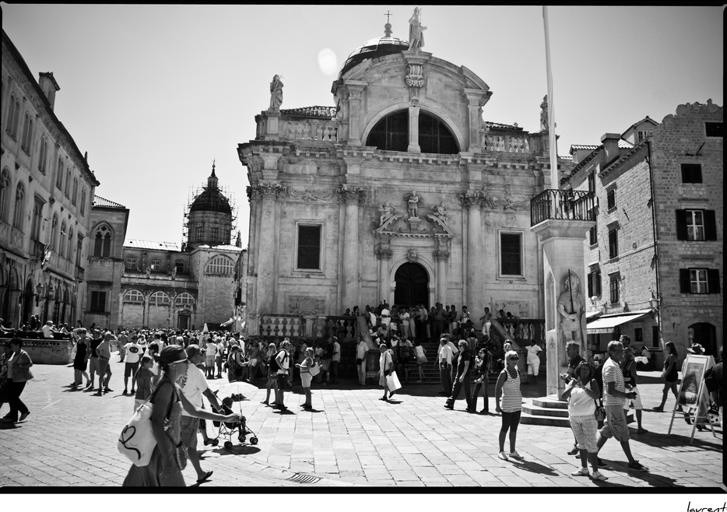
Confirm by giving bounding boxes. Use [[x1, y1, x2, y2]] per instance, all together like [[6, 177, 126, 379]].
[[268, 350, 287, 374]]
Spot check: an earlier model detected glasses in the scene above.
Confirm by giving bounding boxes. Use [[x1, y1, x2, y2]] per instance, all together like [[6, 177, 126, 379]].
[[171, 357, 189, 364], [509, 357, 518, 360]]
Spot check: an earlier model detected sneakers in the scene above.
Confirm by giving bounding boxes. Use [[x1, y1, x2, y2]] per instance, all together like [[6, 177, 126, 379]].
[[628, 460, 650, 471], [122, 389, 127, 395], [497, 450, 508, 460], [20, 410, 30, 420], [637, 427, 648, 433], [71, 382, 113, 396], [510, 451, 522, 458], [131, 389, 136, 394], [379, 396, 387, 400], [205, 437, 214, 446], [567, 447, 607, 480], [654, 405, 664, 412], [207, 373, 223, 379], [676, 408, 684, 412], [2, 414, 19, 421], [261, 400, 312, 412], [444, 404, 489, 414], [389, 391, 395, 397]]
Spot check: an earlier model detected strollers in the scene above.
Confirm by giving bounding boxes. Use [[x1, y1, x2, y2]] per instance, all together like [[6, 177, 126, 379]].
[[209, 390, 258, 452]]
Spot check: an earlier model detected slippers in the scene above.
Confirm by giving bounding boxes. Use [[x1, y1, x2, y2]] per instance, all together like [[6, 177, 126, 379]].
[[196, 470, 212, 483]]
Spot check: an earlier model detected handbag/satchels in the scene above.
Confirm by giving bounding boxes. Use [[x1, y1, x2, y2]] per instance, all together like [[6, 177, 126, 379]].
[[385, 370, 402, 392], [309, 362, 321, 377], [118, 396, 172, 468], [594, 405, 606, 421], [13, 352, 33, 381]]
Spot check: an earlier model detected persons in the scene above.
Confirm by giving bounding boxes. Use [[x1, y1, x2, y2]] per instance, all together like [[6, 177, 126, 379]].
[[436, 202, 450, 226], [539, 95, 556, 132], [407, 7, 428, 50], [407, 190, 419, 217], [381, 200, 391, 226], [270, 75, 284, 108], [559, 272, 583, 366]]
